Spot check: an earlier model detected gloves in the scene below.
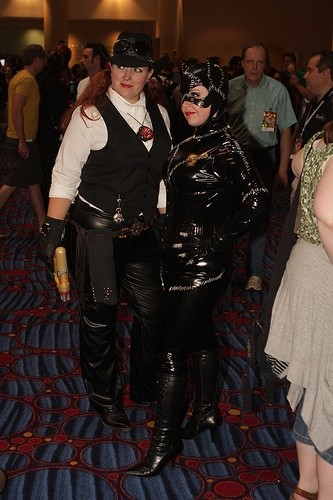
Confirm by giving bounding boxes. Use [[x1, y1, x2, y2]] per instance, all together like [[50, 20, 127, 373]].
[[38, 216, 66, 268]]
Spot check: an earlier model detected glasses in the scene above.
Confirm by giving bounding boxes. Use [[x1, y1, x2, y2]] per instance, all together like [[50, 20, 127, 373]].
[[113, 39, 152, 57]]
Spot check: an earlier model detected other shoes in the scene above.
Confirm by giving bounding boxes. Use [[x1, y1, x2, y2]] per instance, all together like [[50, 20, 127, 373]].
[[289, 485, 317, 500]]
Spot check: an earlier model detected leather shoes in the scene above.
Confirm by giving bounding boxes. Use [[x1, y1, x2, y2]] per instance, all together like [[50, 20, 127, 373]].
[[88, 401, 130, 431], [133, 398, 159, 409]]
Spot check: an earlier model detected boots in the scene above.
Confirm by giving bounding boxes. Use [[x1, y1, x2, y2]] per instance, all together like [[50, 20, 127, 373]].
[[184, 349, 221, 443], [125, 374, 193, 476]]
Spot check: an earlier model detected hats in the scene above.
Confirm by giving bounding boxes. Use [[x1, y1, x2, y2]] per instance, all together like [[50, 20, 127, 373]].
[[106, 32, 162, 78]]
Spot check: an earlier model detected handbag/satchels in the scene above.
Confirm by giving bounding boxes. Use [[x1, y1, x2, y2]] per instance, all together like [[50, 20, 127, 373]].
[[247, 317, 291, 385]]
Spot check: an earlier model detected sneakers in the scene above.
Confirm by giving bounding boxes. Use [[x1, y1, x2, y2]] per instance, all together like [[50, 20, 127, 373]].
[[246, 276, 261, 290]]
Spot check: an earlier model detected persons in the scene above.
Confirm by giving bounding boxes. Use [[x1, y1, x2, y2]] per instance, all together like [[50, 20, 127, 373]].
[[39, 30, 174, 431], [263, 123, 333, 500], [0, 41, 333, 305], [126, 57, 269, 476]]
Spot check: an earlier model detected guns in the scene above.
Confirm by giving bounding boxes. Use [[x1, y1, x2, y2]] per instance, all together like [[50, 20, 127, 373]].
[[53, 247, 71, 302]]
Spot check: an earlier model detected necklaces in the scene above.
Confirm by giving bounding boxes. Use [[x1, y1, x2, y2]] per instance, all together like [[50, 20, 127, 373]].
[[127, 110, 154, 142], [192, 125, 230, 140]]
